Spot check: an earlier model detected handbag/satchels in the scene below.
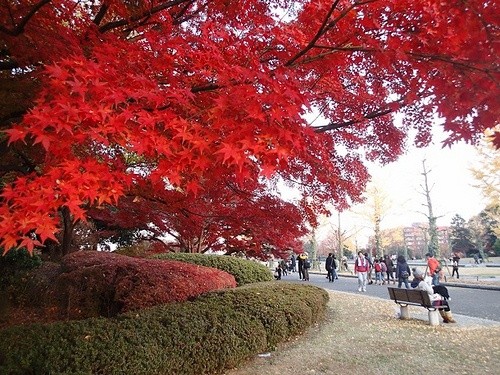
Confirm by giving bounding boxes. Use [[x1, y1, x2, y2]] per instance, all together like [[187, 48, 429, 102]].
[[400, 271, 409, 279]]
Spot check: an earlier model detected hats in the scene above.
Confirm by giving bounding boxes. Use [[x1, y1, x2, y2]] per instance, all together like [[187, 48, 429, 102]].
[[412, 266, 422, 278]]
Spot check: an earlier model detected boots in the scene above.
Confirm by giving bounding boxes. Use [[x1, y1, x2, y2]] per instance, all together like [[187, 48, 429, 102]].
[[439, 310, 446, 322], [444, 311, 456, 323]]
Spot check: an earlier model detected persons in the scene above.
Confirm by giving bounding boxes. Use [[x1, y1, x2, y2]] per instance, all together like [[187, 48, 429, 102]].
[[395, 254, 412, 289], [451, 252, 461, 279], [260, 244, 342, 286], [354, 250, 369, 293], [363, 251, 397, 286], [424, 252, 444, 291], [411, 267, 457, 323]]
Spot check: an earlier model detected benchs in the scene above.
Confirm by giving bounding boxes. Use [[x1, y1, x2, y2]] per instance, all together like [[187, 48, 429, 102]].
[[387, 286, 448, 325]]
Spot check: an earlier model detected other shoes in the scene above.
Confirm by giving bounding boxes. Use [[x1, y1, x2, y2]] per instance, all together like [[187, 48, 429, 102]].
[[326, 277, 338, 282], [298, 278, 310, 281], [357, 280, 396, 292]]
